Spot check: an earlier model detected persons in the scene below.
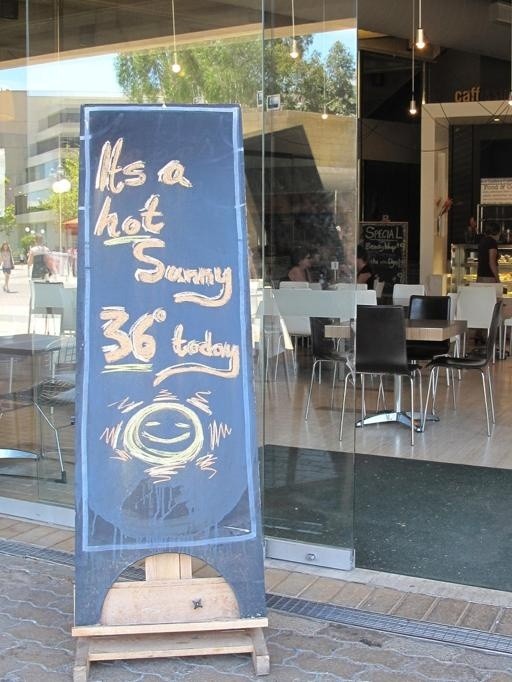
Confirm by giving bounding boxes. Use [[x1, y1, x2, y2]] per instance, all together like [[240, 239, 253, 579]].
[[0, 243, 14, 294], [474, 220, 500, 345], [282, 245, 378, 293], [27, 234, 54, 282]]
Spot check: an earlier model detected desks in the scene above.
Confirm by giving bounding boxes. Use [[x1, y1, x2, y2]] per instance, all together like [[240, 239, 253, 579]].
[[1, 334, 62, 394]]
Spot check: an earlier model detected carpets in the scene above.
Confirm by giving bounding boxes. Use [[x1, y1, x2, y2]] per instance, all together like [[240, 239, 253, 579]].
[[224, 441, 512, 594]]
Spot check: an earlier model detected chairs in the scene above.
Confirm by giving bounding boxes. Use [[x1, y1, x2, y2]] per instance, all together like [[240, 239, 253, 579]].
[[247, 279, 379, 384], [32, 376, 78, 483], [29, 281, 76, 338], [304, 284, 512, 447]]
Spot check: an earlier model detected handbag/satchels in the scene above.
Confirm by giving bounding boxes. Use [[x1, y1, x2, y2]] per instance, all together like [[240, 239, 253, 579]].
[[43, 254, 54, 269], [10, 257, 14, 269]]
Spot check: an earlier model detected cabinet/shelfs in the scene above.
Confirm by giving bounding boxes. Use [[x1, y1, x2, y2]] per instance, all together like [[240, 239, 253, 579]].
[[451, 204, 512, 295]]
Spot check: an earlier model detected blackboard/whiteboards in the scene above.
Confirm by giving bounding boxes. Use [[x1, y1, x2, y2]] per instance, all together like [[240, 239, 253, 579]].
[[358, 221, 408, 297], [74, 103, 267, 627]]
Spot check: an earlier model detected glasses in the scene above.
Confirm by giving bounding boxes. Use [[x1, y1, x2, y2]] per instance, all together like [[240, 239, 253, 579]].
[[303, 256, 313, 260]]
